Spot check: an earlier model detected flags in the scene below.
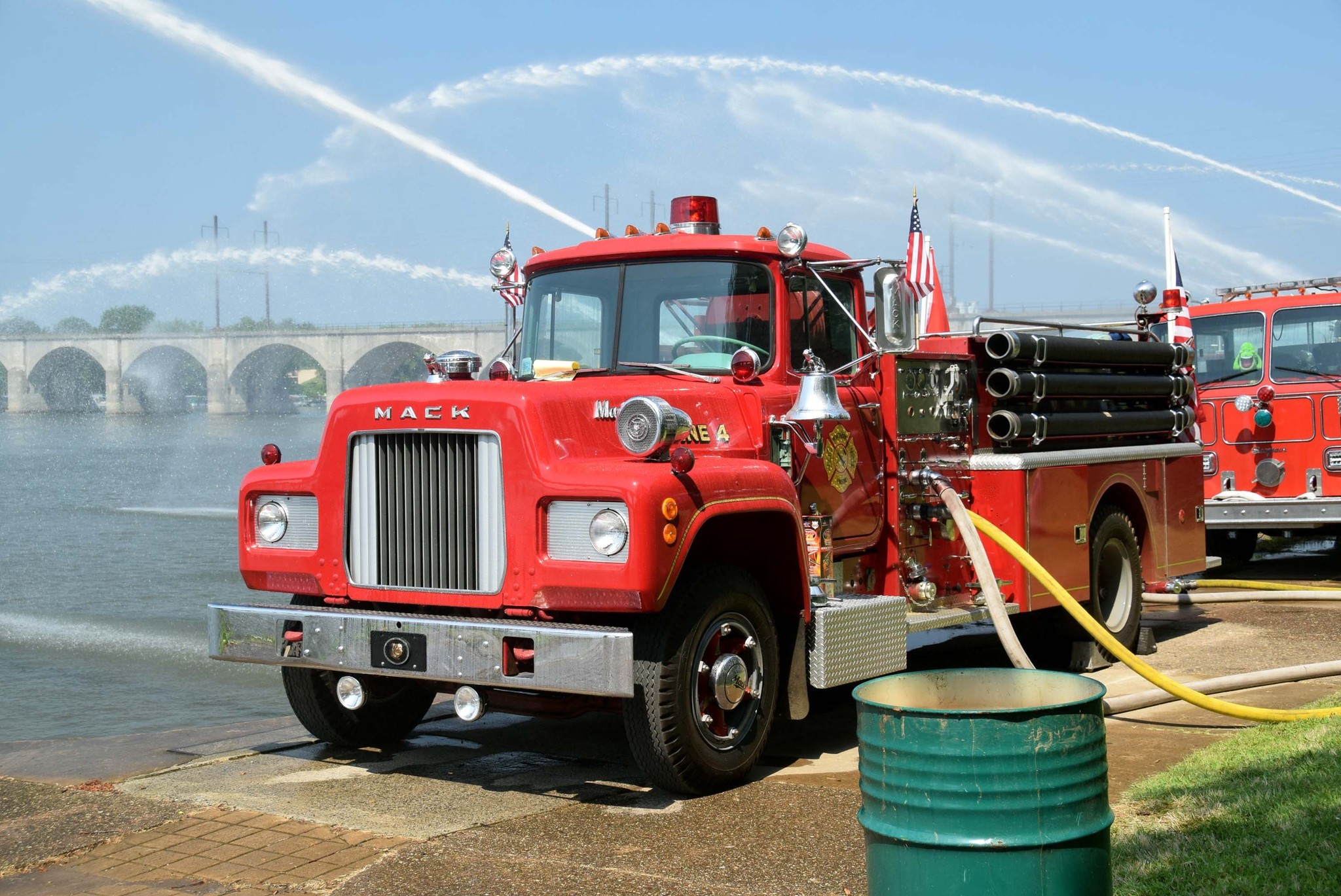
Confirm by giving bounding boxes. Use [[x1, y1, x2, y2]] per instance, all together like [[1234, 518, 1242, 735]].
[[1165, 224, 1207, 446], [906, 198, 952, 339], [498, 233, 526, 307]]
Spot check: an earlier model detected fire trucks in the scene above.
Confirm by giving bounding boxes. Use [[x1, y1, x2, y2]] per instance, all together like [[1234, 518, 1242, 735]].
[[1133, 275, 1341, 572], [204, 191, 1224, 798]]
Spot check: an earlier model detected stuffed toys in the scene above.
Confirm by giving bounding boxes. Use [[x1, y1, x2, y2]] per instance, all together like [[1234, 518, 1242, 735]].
[[1233, 342, 1261, 372]]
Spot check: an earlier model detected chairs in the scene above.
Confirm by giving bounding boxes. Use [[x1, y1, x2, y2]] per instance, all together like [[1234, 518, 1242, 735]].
[[1208, 341, 1341, 371], [700, 316, 805, 366]]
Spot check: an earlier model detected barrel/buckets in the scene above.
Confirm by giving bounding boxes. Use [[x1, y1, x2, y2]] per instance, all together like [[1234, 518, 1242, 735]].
[[850, 666, 1116, 895]]
